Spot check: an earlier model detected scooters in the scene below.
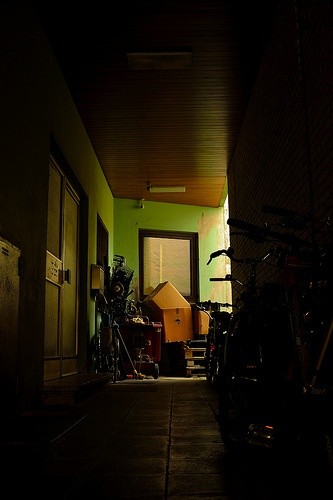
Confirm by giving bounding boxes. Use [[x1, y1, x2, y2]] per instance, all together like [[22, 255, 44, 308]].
[[194, 205, 333, 469]]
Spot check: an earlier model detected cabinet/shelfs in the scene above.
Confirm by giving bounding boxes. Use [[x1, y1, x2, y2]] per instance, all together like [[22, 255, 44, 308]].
[[0, 148, 114, 407]]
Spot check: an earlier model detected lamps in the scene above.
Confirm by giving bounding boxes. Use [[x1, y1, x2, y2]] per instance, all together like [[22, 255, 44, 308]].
[[126, 46, 193, 70], [149, 186, 186, 192]]
